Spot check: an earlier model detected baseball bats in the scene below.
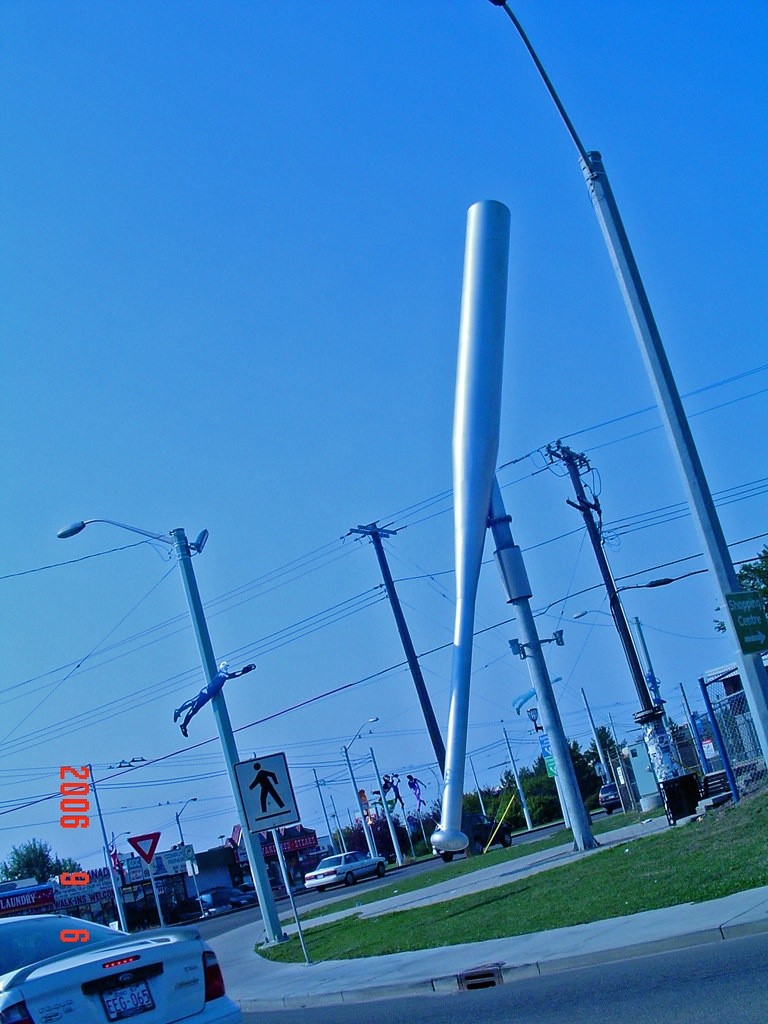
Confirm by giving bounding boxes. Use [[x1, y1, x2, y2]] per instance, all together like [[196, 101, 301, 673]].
[[430, 198, 513, 852]]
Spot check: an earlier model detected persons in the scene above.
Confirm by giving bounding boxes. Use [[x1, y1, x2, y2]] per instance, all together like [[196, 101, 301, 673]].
[[371, 772, 427, 812], [174, 661, 256, 737]]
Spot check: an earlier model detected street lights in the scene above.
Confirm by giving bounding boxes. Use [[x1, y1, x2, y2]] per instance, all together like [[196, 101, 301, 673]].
[[572, 607, 686, 778], [330, 814, 344, 853], [217, 835, 225, 845], [341, 716, 381, 857], [174, 798, 208, 918], [55, 515, 288, 947], [102, 831, 132, 868]]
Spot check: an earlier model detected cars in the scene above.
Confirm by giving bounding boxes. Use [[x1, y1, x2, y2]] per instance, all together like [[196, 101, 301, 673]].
[[173, 881, 286, 922], [304, 850, 388, 893], [0, 913, 249, 1024]]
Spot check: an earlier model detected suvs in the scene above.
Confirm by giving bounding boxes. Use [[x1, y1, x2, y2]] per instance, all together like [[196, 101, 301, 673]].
[[597, 782, 625, 816], [428, 813, 514, 864]]
